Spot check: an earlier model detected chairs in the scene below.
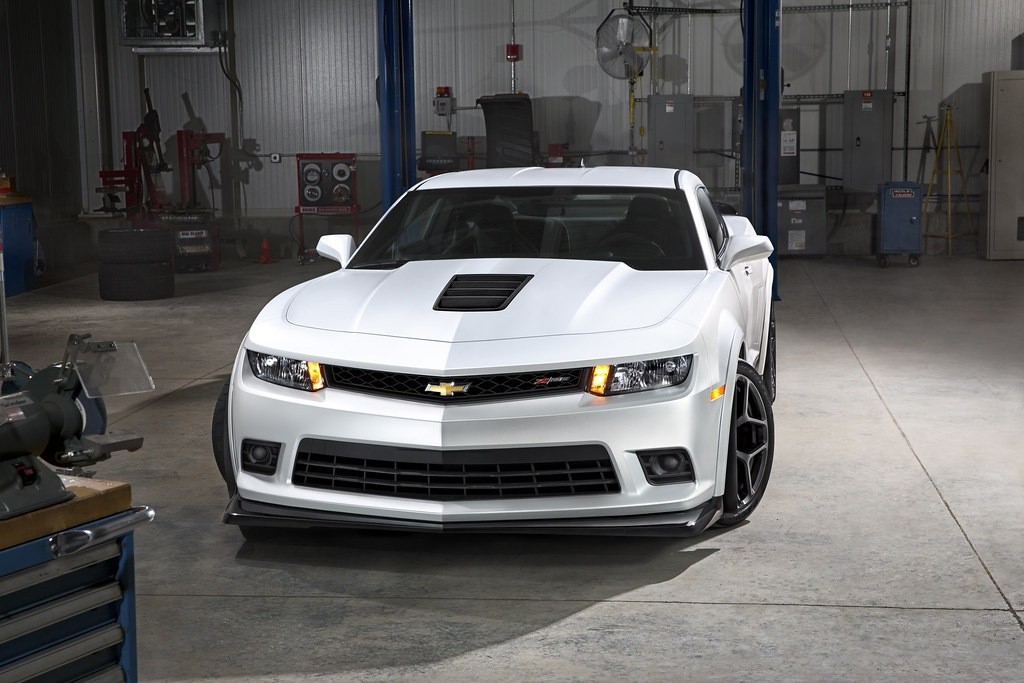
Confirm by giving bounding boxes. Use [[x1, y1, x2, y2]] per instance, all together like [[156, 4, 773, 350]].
[[465, 204, 537, 254], [593, 195, 692, 262]]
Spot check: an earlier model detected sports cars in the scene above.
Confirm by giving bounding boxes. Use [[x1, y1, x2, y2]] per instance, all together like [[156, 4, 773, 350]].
[[210, 166, 783, 540]]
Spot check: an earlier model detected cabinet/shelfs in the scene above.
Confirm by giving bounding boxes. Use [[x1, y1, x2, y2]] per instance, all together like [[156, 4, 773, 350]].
[[646, 94, 693, 172], [983, 71, 1024, 259], [778, 90, 924, 265], [0, 196, 36, 298]]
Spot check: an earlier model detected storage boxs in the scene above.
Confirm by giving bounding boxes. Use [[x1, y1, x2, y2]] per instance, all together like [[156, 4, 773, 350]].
[[0, 176, 17, 195]]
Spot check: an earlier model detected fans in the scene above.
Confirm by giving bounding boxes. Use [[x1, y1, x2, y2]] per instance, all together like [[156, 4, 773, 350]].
[[596, 6, 654, 80]]
